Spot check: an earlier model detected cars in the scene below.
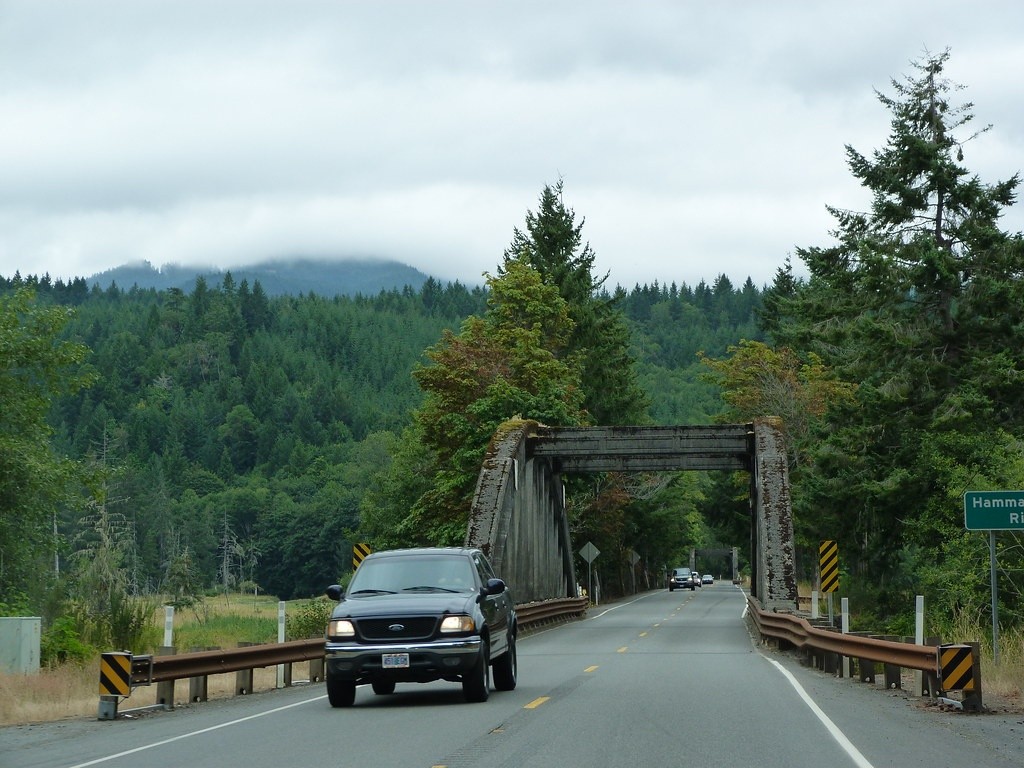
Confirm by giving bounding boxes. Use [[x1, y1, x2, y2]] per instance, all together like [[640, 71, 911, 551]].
[[690, 572, 701, 584], [327, 546, 518, 702], [705, 575, 715, 584], [671, 568, 695, 591]]
